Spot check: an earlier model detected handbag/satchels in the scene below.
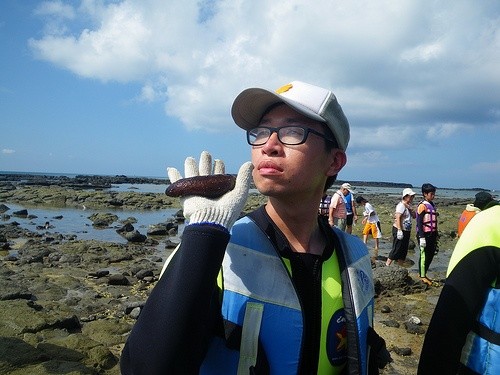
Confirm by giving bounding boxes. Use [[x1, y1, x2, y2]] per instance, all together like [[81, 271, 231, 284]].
[[409, 207, 416, 219]]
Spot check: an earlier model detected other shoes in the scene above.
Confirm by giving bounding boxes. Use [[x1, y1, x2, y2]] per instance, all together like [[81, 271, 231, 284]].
[[422, 277, 433, 286]]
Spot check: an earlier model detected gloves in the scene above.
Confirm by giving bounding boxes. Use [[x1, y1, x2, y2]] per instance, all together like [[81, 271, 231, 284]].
[[397, 230, 403, 240], [420, 237, 426, 248]]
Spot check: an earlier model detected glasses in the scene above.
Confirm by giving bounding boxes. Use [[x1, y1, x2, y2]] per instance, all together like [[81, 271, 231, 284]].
[[407, 195, 414, 199], [247, 126, 337, 146]]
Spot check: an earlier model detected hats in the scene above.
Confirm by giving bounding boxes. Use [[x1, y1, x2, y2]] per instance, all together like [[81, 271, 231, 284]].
[[476, 192, 492, 205], [167, 152, 255, 234], [403, 188, 416, 199], [342, 183, 353, 193], [231, 81, 350, 151], [356, 196, 365, 202]]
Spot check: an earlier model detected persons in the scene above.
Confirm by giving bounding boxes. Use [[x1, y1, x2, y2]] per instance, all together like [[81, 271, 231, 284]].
[[117, 80, 375, 375], [321, 183, 500, 375]]
